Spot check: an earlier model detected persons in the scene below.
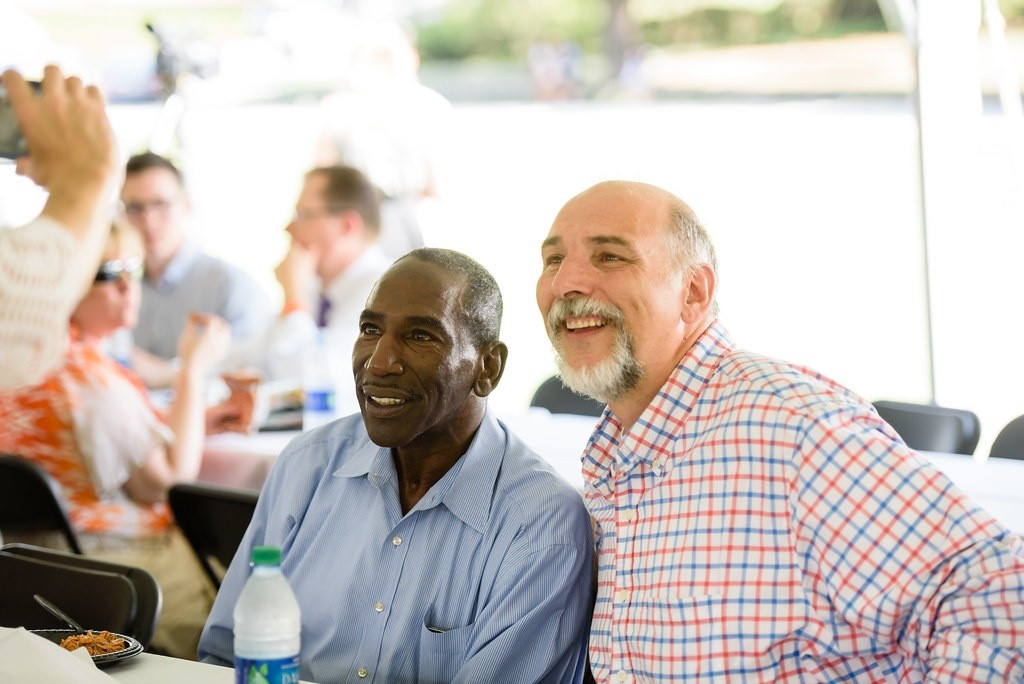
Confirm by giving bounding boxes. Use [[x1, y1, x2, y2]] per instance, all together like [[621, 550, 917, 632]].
[[263, 166, 415, 392], [2, 216, 238, 662], [1, 65, 127, 397], [192, 247, 598, 683], [116, 152, 262, 392], [534, 177, 1024, 684]]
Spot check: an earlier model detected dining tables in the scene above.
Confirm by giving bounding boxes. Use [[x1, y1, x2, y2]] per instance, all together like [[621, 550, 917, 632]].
[[1, 622, 323, 684]]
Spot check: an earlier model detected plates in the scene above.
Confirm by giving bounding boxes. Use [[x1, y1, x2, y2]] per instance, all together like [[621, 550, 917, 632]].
[[28, 629, 144, 667]]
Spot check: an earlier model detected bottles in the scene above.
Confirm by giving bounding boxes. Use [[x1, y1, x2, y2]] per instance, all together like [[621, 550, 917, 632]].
[[300, 366, 333, 431], [232, 546, 301, 683]]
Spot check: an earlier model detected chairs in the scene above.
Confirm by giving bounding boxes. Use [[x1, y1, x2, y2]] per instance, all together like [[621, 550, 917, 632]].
[[0, 454, 86, 558], [870, 402, 984, 457], [168, 483, 262, 597], [988, 415, 1023, 461], [0, 543, 162, 653]]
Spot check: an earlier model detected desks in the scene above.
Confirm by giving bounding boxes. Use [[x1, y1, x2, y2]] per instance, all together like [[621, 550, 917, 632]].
[[198, 403, 1024, 537]]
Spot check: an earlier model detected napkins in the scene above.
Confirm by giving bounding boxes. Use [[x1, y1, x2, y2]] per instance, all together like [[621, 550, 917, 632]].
[[0, 626, 116, 684]]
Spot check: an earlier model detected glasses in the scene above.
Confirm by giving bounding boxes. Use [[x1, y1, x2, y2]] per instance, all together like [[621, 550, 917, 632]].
[[94, 258, 146, 286]]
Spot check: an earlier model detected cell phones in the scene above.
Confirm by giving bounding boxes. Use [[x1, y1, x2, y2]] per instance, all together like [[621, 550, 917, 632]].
[[1, 78, 42, 160]]
